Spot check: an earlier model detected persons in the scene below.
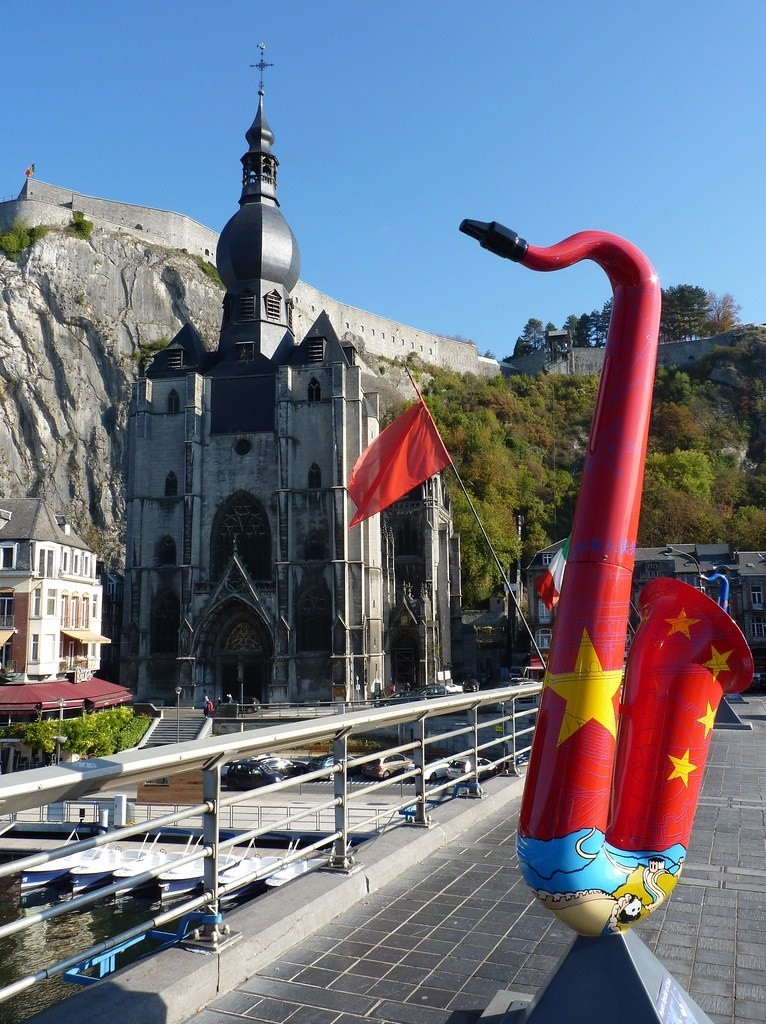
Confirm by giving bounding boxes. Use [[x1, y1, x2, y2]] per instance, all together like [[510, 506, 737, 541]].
[[227, 694, 232, 703], [203, 696, 209, 718], [217, 696, 221, 705], [390, 682, 411, 693]]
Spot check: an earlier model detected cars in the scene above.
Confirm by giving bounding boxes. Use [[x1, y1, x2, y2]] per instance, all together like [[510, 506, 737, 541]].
[[402, 757, 450, 782], [231, 697, 261, 713], [373, 691, 427, 709], [223, 761, 284, 789], [417, 687, 452, 698], [445, 683, 463, 694], [460, 677, 481, 694]]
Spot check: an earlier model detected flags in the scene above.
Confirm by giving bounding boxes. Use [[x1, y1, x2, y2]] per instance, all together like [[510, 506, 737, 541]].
[[349, 399, 451, 528], [533, 532, 572, 610], [399, 604, 410, 620]]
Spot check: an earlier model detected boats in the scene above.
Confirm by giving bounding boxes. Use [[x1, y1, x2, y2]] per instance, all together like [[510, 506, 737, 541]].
[[156, 835, 256, 899], [17, 840, 110, 891], [111, 833, 204, 894], [198, 854, 282, 899], [68, 830, 162, 895], [263, 838, 352, 891]]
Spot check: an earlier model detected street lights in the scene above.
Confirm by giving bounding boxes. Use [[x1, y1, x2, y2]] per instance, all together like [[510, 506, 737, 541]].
[[55, 697, 67, 763], [666, 544, 704, 593], [174, 686, 183, 740]]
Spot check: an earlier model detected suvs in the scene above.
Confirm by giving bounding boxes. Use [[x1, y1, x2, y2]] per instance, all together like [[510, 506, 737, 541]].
[[256, 757, 308, 779], [361, 752, 413, 781], [306, 753, 355, 782], [446, 756, 495, 779], [509, 665, 524, 680]]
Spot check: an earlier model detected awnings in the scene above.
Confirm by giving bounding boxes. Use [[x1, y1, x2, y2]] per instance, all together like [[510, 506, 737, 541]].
[[62, 629, 111, 643], [0, 630, 14, 647]]
